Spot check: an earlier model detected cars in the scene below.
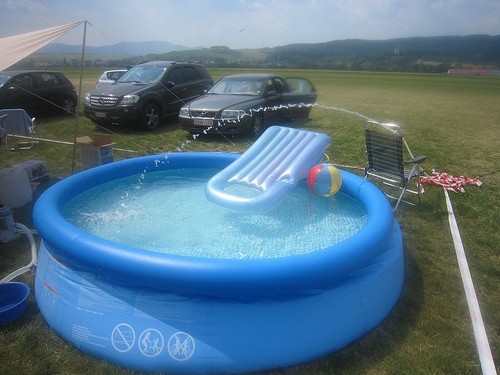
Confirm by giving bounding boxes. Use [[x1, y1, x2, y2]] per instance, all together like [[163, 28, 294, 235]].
[[96, 70, 128, 89], [179, 73, 317, 137], [0, 70, 78, 116]]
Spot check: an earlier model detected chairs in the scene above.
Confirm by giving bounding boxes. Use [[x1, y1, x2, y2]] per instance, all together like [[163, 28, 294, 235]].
[[0, 109, 42, 151], [358, 129, 428, 213]]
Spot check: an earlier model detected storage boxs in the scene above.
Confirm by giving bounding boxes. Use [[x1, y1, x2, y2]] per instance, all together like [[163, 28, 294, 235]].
[[76, 134, 115, 171]]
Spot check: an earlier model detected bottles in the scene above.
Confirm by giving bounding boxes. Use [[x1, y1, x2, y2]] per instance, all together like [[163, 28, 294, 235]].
[[0, 207, 16, 244]]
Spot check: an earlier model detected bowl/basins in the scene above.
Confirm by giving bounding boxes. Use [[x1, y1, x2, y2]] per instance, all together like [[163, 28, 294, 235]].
[[0, 281, 31, 325]]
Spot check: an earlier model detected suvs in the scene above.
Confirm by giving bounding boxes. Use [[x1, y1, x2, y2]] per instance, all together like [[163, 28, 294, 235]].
[[83, 61, 214, 131]]
